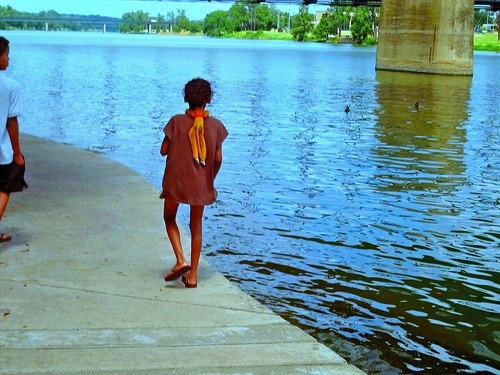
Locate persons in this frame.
[159,78,229,287]
[0,36,28,244]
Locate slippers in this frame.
[181,271,198,288]
[164,264,192,282]
[1,233,12,243]
